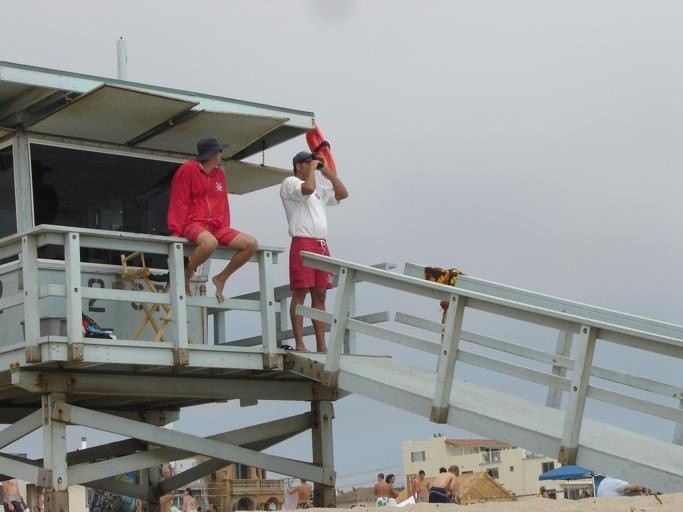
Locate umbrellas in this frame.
[537,464,595,498]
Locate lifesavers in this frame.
[306,127,336,179]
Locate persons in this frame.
[279,151,350,352]
[134,496,142,511]
[538,485,549,499]
[427,464,461,504]
[1,478,26,512]
[372,473,389,507]
[167,136,258,303]
[159,491,177,511]
[438,466,447,473]
[411,469,432,502]
[181,486,198,512]
[285,478,314,508]
[384,472,400,507]
[595,476,643,496]
[161,462,171,480]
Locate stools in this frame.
[119,251,197,343]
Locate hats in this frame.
[291,151,318,166]
[192,137,229,162]
